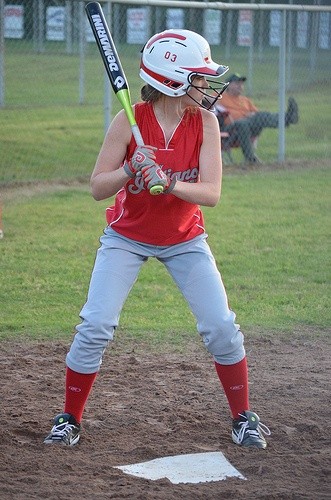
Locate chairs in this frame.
[221,131,240,164]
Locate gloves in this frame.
[123,144,158,177]
[136,163,176,194]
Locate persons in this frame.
[211,73,298,167]
[42,29,271,450]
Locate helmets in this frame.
[139,28,229,97]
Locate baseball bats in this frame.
[84,1,164,196]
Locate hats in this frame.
[226,74,247,83]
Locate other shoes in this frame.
[286,98,298,126]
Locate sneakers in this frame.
[42,413,80,447]
[230,411,271,448]
